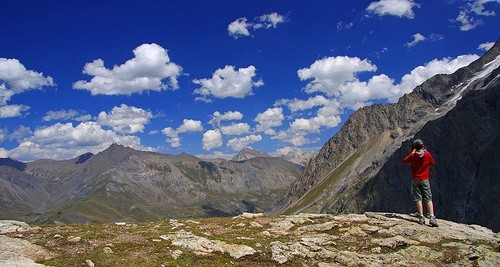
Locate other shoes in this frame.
[419,215,425,225]
[410,212,418,217]
[429,215,439,227]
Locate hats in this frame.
[413,139,423,147]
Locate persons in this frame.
[402,139,439,226]
